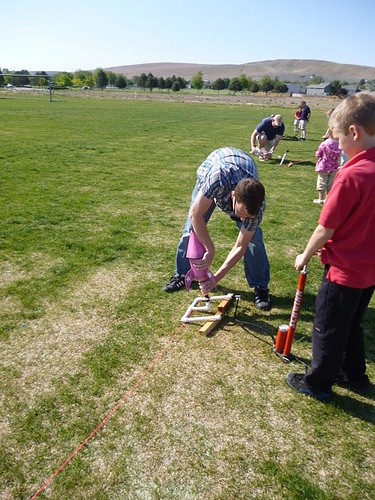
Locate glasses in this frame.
[234,198,258,220]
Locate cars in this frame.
[82,86,93,89]
[5,83,14,89]
[25,85,32,88]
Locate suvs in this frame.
[48,82,70,90]
[105,85,119,89]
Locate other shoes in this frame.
[313,199,326,204]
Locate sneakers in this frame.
[164,276,191,293]
[335,374,370,391]
[255,290,271,310]
[288,373,332,400]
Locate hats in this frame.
[274,115,283,126]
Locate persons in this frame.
[251,114,285,158]
[313,128,341,204]
[327,108,346,169]
[294,100,311,140]
[163,148,272,311]
[285,90,375,400]
